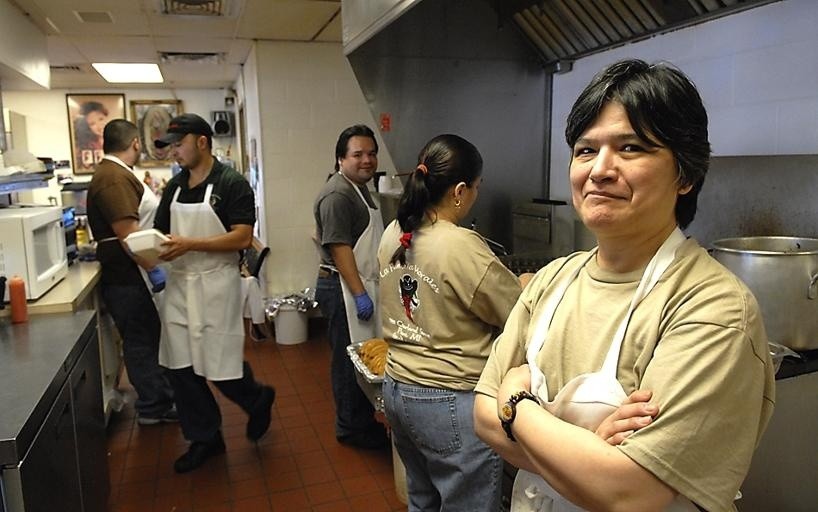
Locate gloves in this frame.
[146,265,166,293]
[353,290,374,322]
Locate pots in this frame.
[707,236,818,350]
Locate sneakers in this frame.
[245,386,275,442]
[135,403,184,426]
[338,422,390,448]
[173,430,227,474]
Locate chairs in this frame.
[237,235,269,343]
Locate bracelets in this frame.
[496,389,541,442]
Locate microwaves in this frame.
[0,208,68,303]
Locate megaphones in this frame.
[222,88,236,107]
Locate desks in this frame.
[0,259,125,430]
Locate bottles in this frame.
[144,171,152,189]
[0,274,28,324]
[74,217,89,251]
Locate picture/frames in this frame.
[129,100,185,169]
[66,93,126,175]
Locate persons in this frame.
[376,131,538,511]
[72,101,115,171]
[468,57,777,511]
[310,123,391,451]
[149,111,276,474]
[83,117,179,427]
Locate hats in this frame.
[154,114,214,149]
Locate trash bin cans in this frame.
[275,304,308,345]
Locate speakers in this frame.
[211,111,234,137]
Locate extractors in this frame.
[497,0,818,73]
